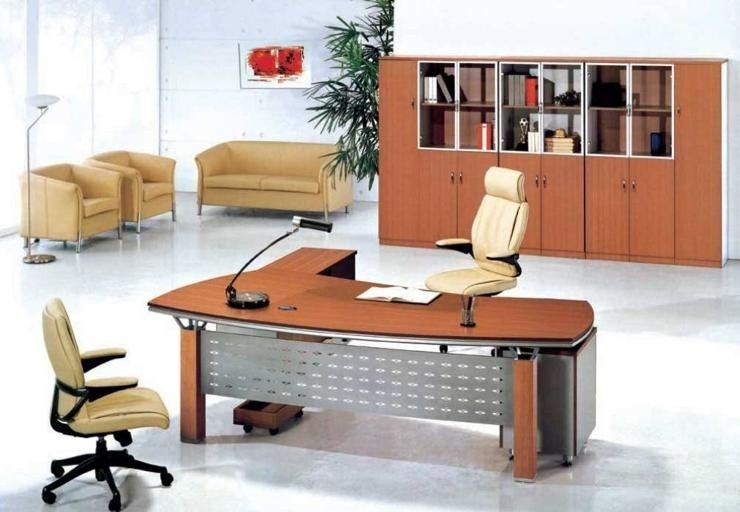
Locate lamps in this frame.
[224,213,333,309]
[22,93,60,265]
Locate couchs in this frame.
[193,139,354,221]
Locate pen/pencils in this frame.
[278,306,298,310]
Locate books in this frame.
[423,73,581,153]
[354,285,442,305]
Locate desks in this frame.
[148,246,594,486]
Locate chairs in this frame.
[424,166,531,298]
[41,297,173,512]
[18,149,177,254]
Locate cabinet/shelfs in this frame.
[418,56,673,159]
[495,326,598,469]
[673,56,730,267]
[417,147,675,264]
[377,55,417,246]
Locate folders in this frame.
[423,74,454,105]
